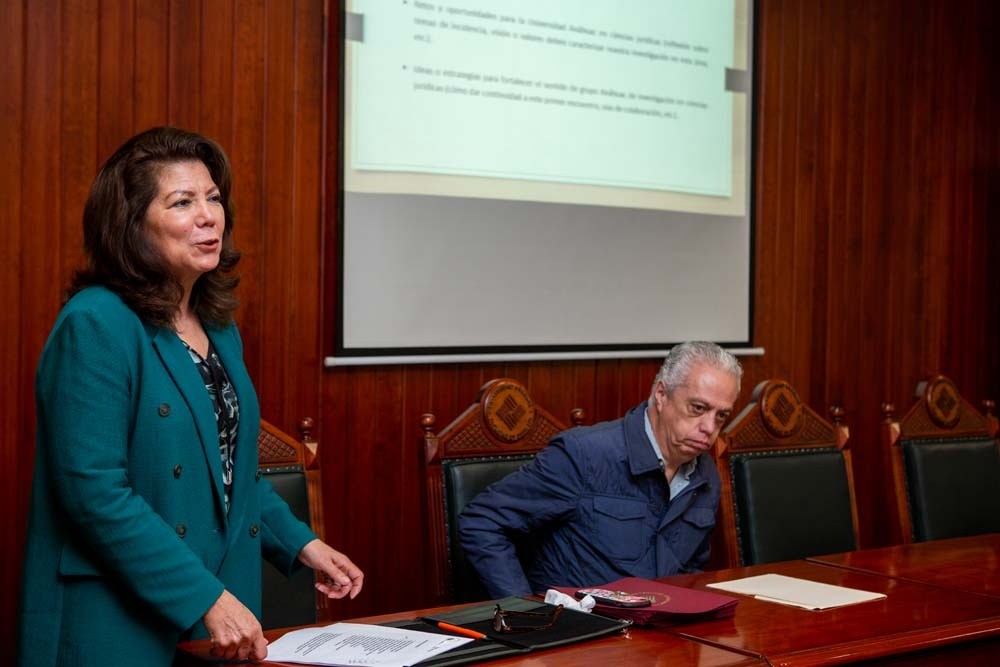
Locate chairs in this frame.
[420,378,586,607]
[259,414,332,630]
[715,379,860,567]
[881,374,1000,545]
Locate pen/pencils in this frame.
[437,622,488,639]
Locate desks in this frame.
[179,534,1000,667]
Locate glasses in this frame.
[493,603,564,632]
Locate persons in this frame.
[455,340,742,600]
[10,127,364,667]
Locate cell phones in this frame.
[574,588,651,608]
